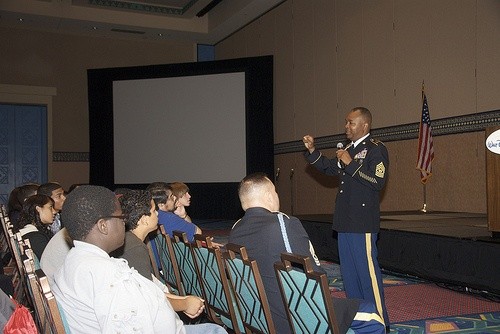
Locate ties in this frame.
[348,145,354,152]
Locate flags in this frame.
[415,93,437,185]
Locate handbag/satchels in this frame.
[4,306,36,333]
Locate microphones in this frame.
[275,167,280,180]
[337,143,343,168]
[290,169,294,179]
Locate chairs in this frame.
[0,205,344,334]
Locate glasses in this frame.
[95,214,129,224]
[157,193,175,204]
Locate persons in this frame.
[50,186,228,334]
[228,172,360,334]
[109,190,205,319]
[17,184,38,206]
[303,107,390,334]
[7,187,21,231]
[40,227,75,292]
[147,182,202,274]
[169,182,224,249]
[18,195,56,262]
[114,188,130,199]
[37,183,66,235]
